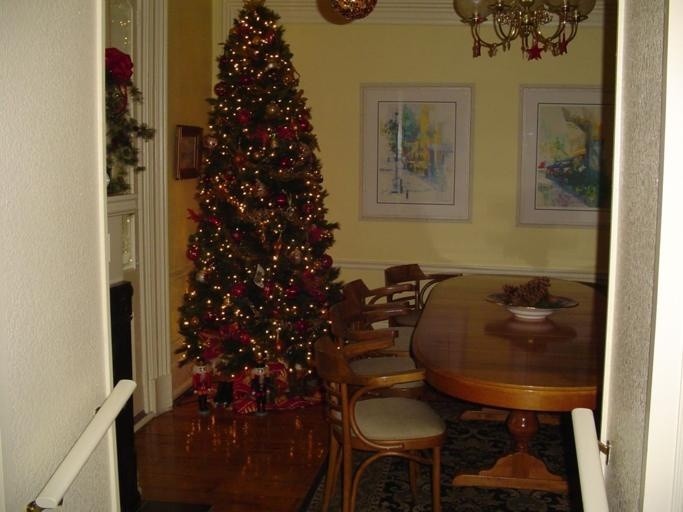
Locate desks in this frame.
[410,273,605,497]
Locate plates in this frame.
[484,294,579,318]
[483,316,577,343]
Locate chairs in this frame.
[311,260,461,511]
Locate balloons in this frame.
[328,0,377,20]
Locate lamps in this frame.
[450,0,595,63]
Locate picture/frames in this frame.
[172,124,204,181]
[514,81,612,230]
[356,82,474,224]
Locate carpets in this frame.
[295,398,584,511]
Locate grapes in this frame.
[497,275,554,312]
